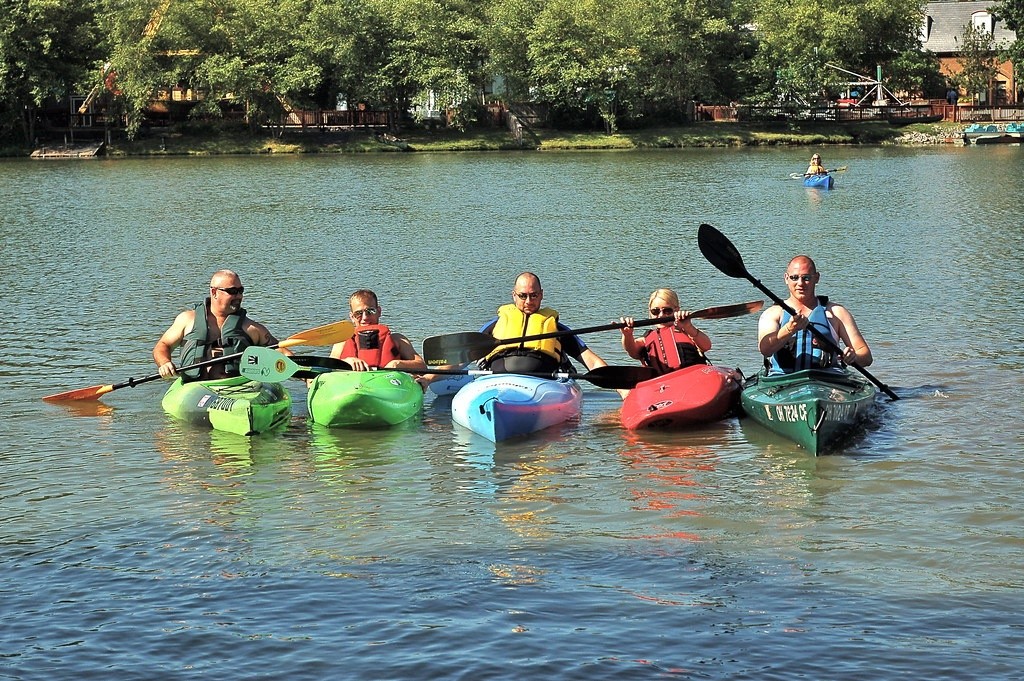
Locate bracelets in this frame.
[784,324,795,336]
[689,328,699,337]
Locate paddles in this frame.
[41,319,355,401]
[286,356,659,389]
[697,224,900,400]
[423,301,766,366]
[239,346,475,396]
[789,166,847,180]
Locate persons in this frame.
[152,268,317,388]
[757,254,873,375]
[609,289,712,369]
[327,289,429,375]
[414,272,631,401]
[804,153,829,178]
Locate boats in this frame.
[620,364,742,432]
[451,372,584,445]
[958,122,1024,144]
[306,370,423,430]
[429,376,475,396]
[162,376,293,437]
[804,174,834,189]
[741,363,879,459]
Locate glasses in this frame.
[786,275,816,281]
[514,292,541,300]
[649,307,677,315]
[352,307,378,319]
[813,158,820,160]
[212,286,244,296]
